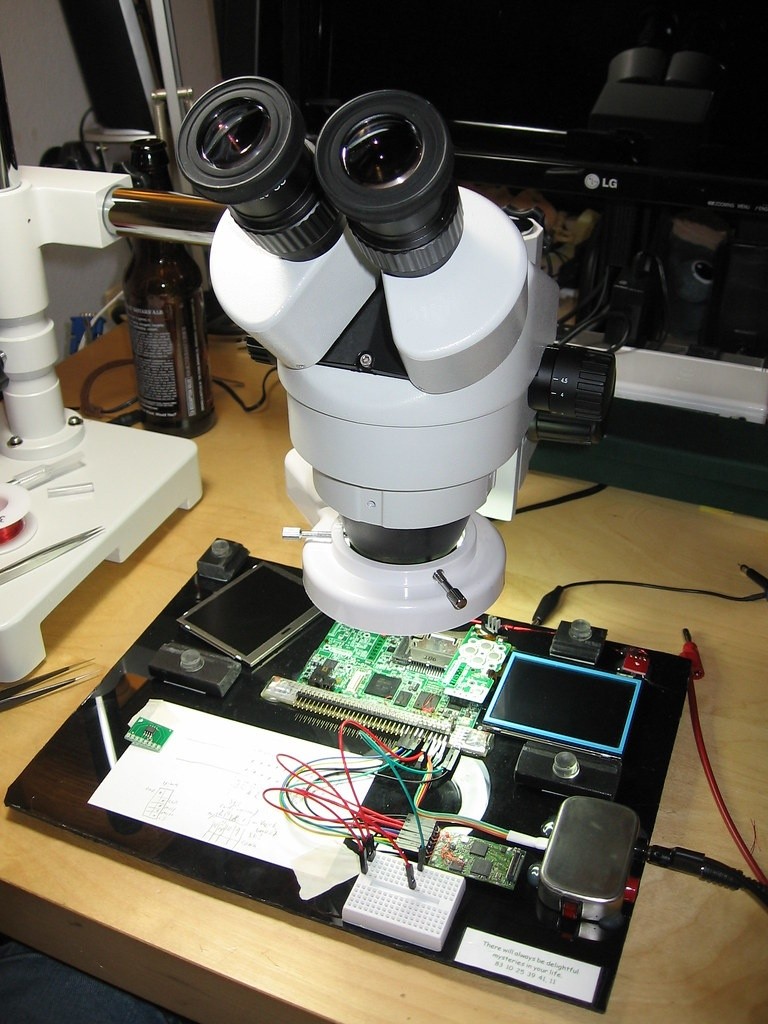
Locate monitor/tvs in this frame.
[210,0,768,222]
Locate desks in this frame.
[0,318,768,1023]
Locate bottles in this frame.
[123,140,217,441]
